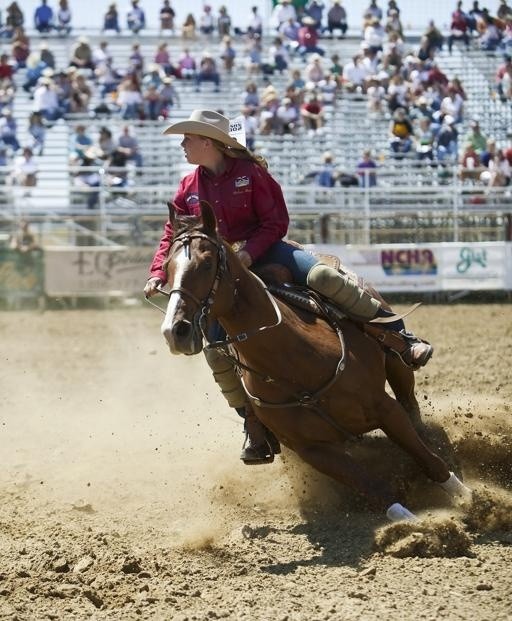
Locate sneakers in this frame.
[400,327,435,368]
[239,415,280,463]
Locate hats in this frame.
[162,106,250,153]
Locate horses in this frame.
[160,200,474,526]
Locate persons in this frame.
[0,0,511,245]
[8,218,44,279]
[141,105,434,465]
